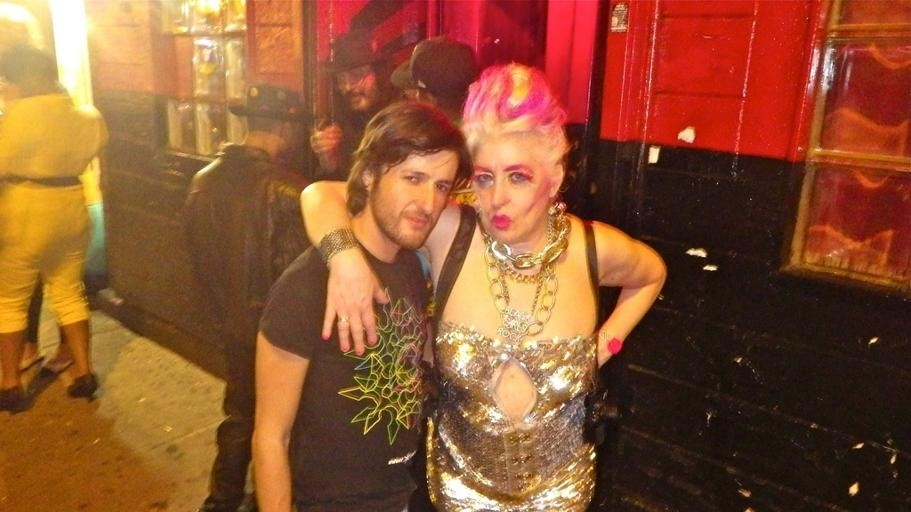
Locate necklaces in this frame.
[474,202,574,343]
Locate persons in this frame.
[242,100,474,511]
[385,36,482,129]
[297,59,668,512]
[0,47,113,420]
[307,26,414,183]
[175,83,324,512]
[0,16,79,378]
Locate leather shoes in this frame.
[40,352,74,374]
[19,347,48,372]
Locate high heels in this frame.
[65,378,99,400]
[0,385,22,411]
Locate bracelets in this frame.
[596,325,625,356]
[315,223,362,273]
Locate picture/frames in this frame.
[780,3,911,288]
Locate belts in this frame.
[8,175,86,189]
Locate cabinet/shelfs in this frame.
[85,2,306,373]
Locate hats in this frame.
[228,82,314,121]
[318,35,386,74]
[389,36,480,98]
